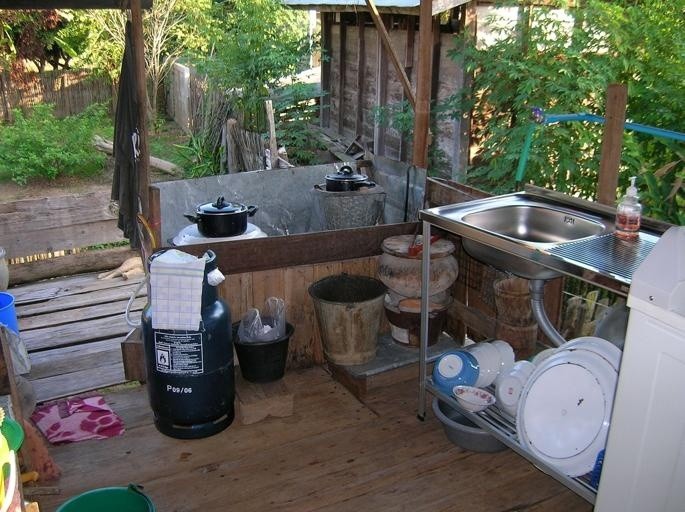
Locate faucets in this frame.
[513,108,546,182]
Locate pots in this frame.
[183,196,258,237]
[314,165,376,192]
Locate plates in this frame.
[495,361,538,417]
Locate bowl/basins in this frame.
[454,385,496,413]
[432,339,516,391]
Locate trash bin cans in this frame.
[232,315,295,383]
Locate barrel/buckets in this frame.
[309,273,387,366]
[53,481,156,512]
[0,430,17,512]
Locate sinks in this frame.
[460,202,606,246]
